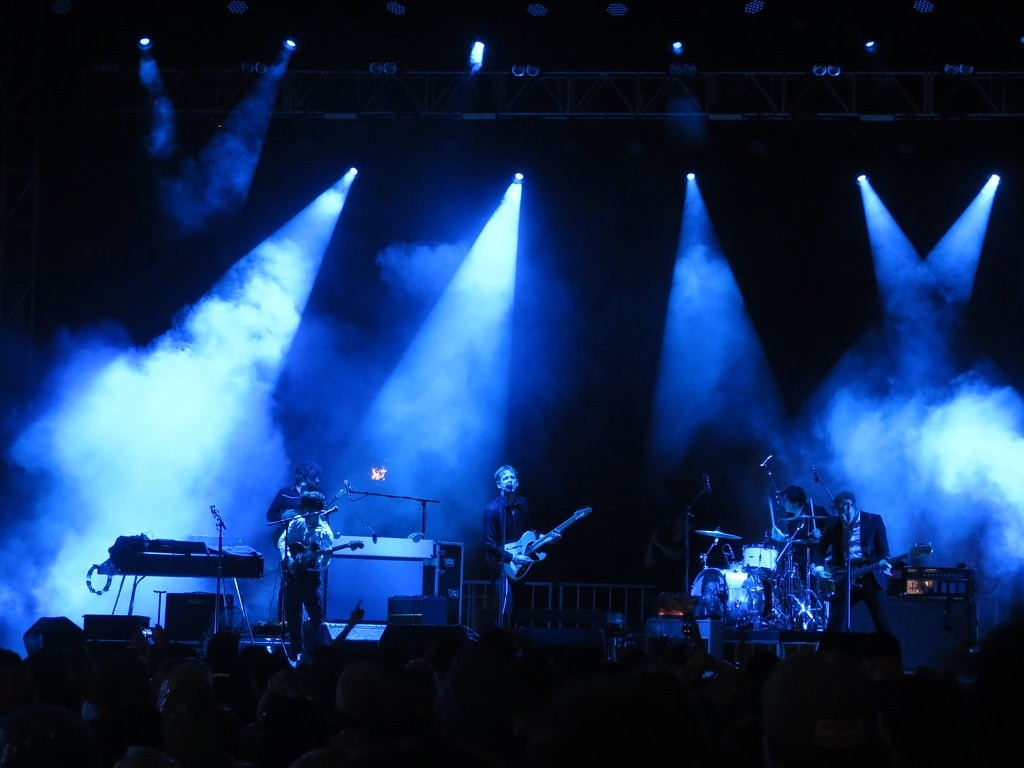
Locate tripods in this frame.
[758,519,826,631]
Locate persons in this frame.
[266,462,329,625]
[278,492,334,661]
[483,465,562,631]
[0,577,1024,768]
[817,491,890,631]
[781,485,832,580]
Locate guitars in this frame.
[282,540,365,575]
[273,485,353,548]
[819,541,934,600]
[502,505,593,582]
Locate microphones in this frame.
[209,504,217,518]
[760,455,772,467]
[846,500,853,505]
[321,506,338,516]
[812,465,819,483]
[505,483,512,489]
[344,479,351,498]
[727,544,735,558]
[706,476,712,494]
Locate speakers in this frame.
[517,626,607,659]
[165,594,234,641]
[814,633,903,678]
[307,622,387,646]
[24,616,90,657]
[376,598,469,656]
[646,618,724,661]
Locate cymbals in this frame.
[791,537,819,545]
[693,529,742,540]
[778,514,830,521]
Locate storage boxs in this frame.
[83,615,149,640]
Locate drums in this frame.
[690,567,764,626]
[771,562,799,579]
[742,544,780,575]
[728,561,743,570]
